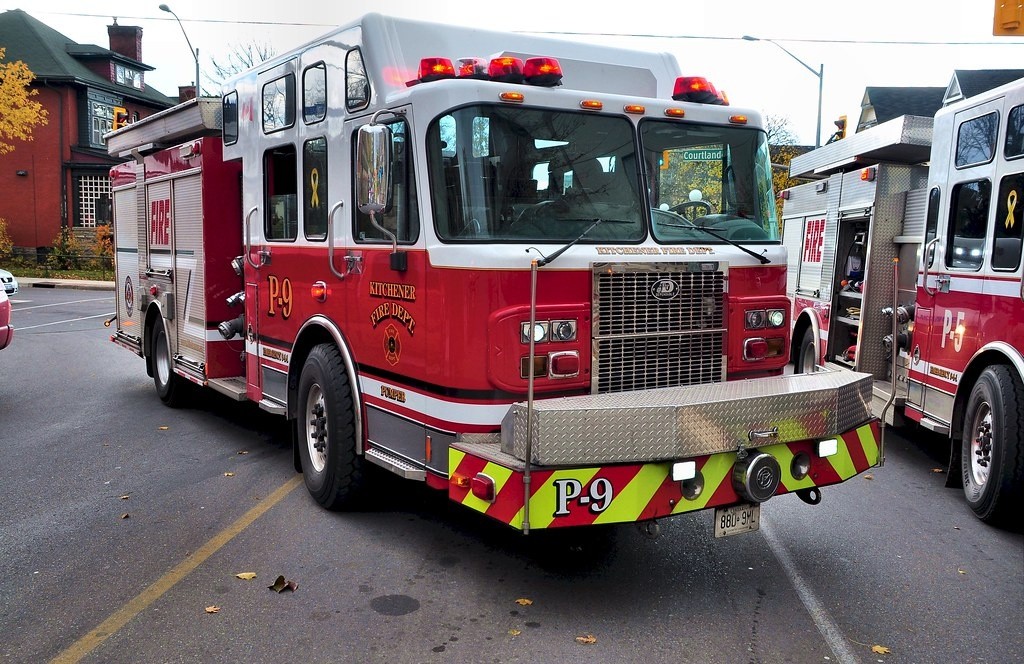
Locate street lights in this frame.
[741,36,824,150]
[159,4,200,98]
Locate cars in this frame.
[0,269,18,297]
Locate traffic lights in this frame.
[833,116,846,141]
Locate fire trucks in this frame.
[101,13,888,535]
[779,74,1024,530]
